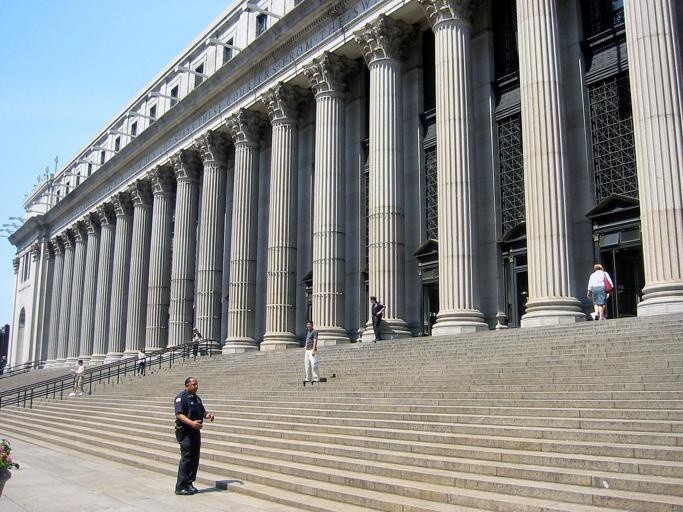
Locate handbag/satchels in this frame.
[605,277,612,293]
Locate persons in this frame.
[135,348,146,377]
[370,295,385,342]
[586,264,614,320]
[190,327,202,359]
[302,321,320,382]
[173,377,214,495]
[68,360,85,397]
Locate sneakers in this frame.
[69,392,85,397]
[591,312,606,320]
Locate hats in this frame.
[594,263,602,268]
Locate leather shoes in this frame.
[175,484,197,495]
